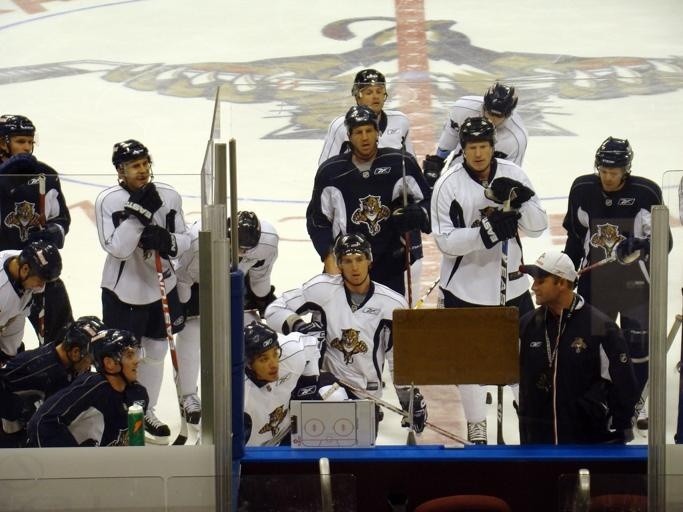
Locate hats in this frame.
[519,251,577,283]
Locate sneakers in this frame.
[637,407,648,431]
[180,395,201,424]
[142,413,171,437]
[467,420,487,442]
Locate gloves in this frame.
[490,177,536,210]
[479,208,522,250]
[0,152,36,173]
[291,375,318,399]
[616,237,650,266]
[393,205,430,233]
[138,225,177,259]
[294,320,324,334]
[124,182,163,227]
[422,154,446,188]
[29,223,64,249]
[401,388,429,434]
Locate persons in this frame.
[0,115,280,446]
[239,70,673,448]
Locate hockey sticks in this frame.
[153,253,188,446]
[401,129,412,312]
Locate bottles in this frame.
[127,404,144,445]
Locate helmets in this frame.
[351,69,386,97]
[227,211,261,251]
[345,105,378,133]
[459,116,496,148]
[62,315,144,369]
[334,233,372,260]
[0,114,35,137]
[484,83,518,118]
[112,140,148,163]
[245,321,278,357]
[595,136,634,169]
[18,240,62,282]
